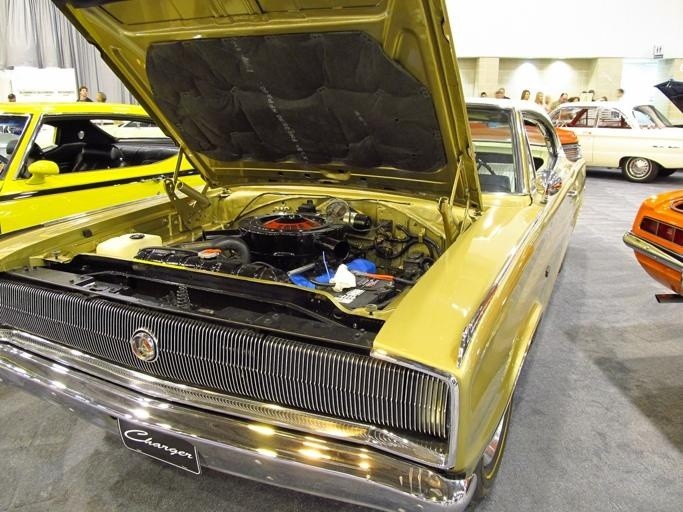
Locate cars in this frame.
[0,122,24,165]
[651,78,683,128]
[467,118,580,162]
[0,100,208,254]
[547,102,682,183]
[99,119,177,147]
[0,3,584,512]
[620,190,682,304]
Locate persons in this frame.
[76,86,92,101]
[96,91,106,102]
[480,88,624,113]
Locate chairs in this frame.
[4,138,45,179]
[71,143,127,172]
